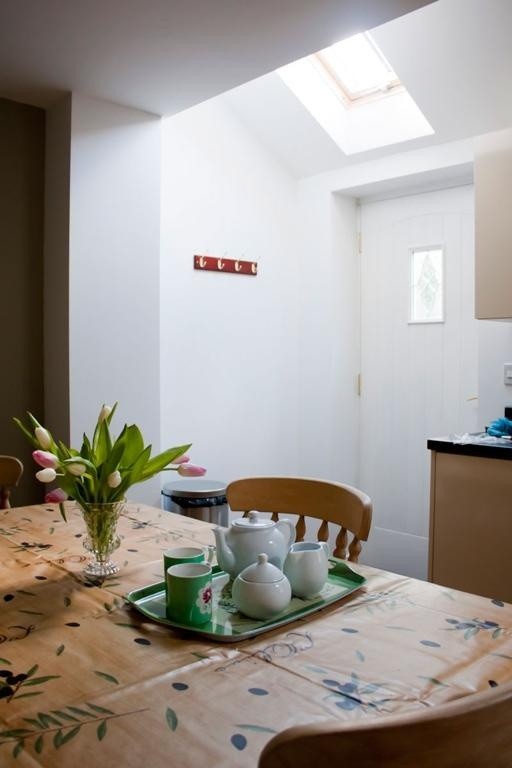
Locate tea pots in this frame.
[212,510,295,576]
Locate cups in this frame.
[162,544,216,628]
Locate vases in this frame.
[75,497,127,576]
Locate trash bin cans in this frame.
[160,479,229,528]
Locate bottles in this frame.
[284,540,331,597]
[230,554,291,620]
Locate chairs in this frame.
[255,685,509,768]
[227,473,370,570]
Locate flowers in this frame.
[13,403,209,554]
